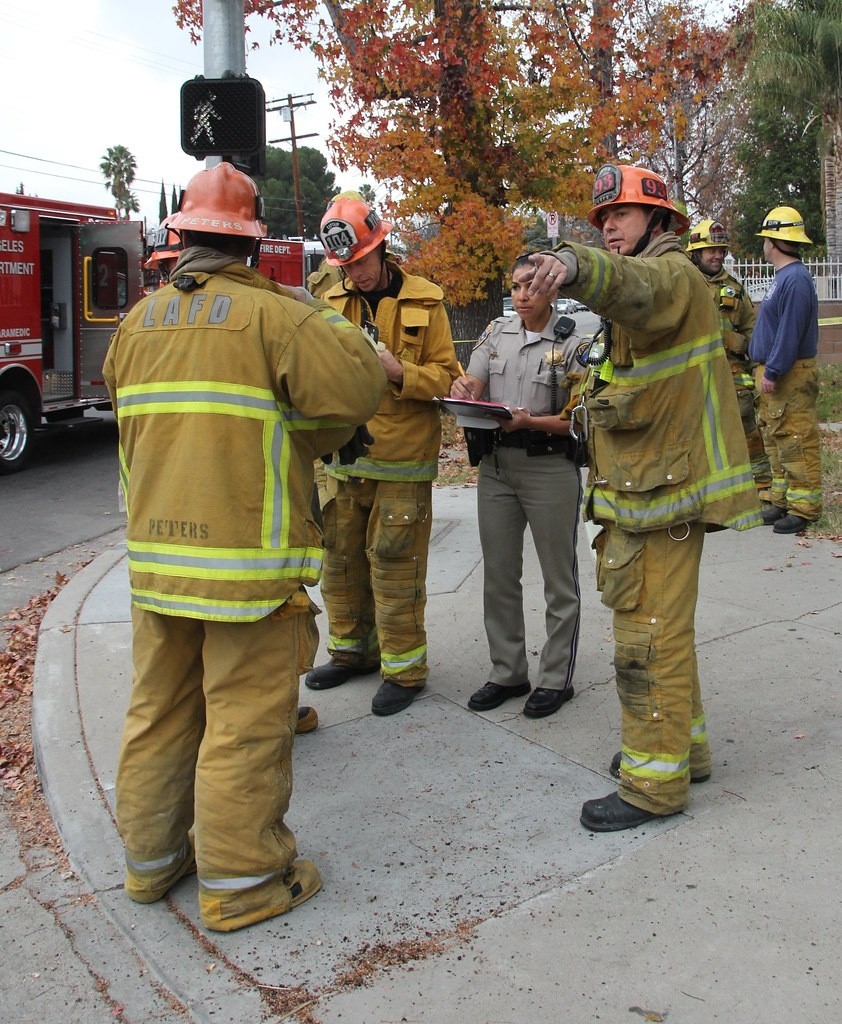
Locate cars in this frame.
[504,297,517,317]
[551,298,590,314]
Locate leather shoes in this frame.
[760,506,788,525]
[371,679,425,716]
[580,791,684,831]
[305,655,381,690]
[609,751,711,784]
[523,685,574,718]
[773,514,822,534]
[468,680,531,711]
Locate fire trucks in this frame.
[0,192,156,476]
[257,238,325,288]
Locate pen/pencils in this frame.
[457,361,475,402]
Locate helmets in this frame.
[686,220,732,252]
[755,206,813,245]
[143,212,183,269]
[325,191,366,210]
[587,163,690,236]
[166,162,268,238]
[319,197,394,267]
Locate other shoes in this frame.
[200,859,323,932]
[294,706,318,733]
[125,828,197,904]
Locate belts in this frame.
[495,427,530,450]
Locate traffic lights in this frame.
[180,72,266,158]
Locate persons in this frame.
[450,250,595,719]
[306,190,402,299]
[517,164,765,834]
[685,219,774,511]
[747,206,824,534]
[101,162,390,933]
[304,198,461,717]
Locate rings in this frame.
[547,272,556,280]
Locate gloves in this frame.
[320,425,376,466]
